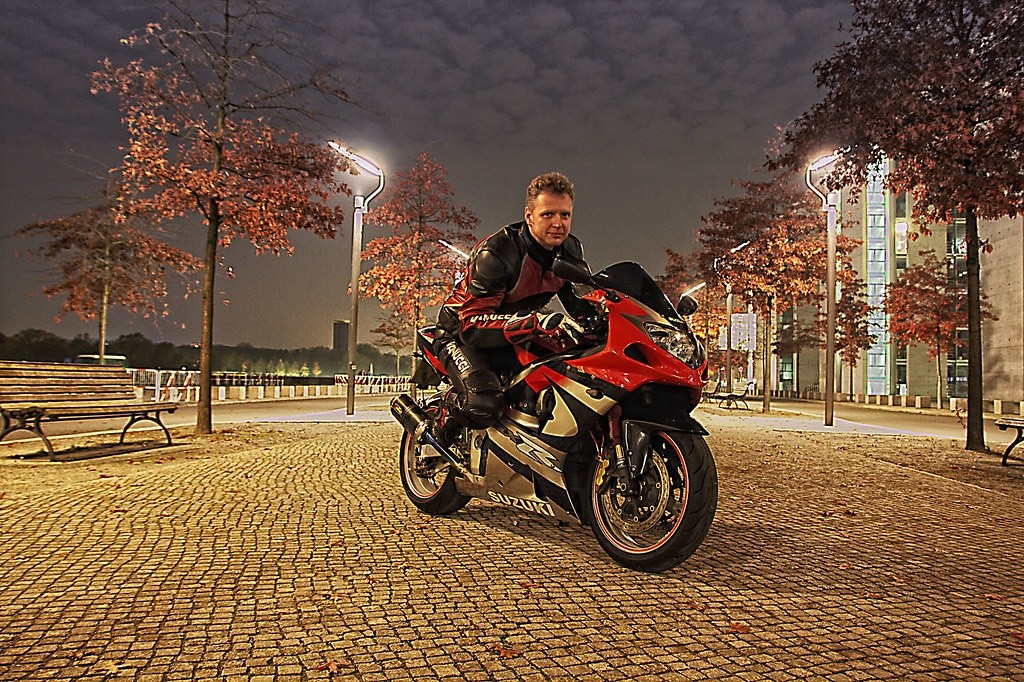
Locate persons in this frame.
[433,172,592,446]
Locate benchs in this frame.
[993,417,1024,466]
[0,359,179,462]
[717,381,754,410]
[700,381,722,404]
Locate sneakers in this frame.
[430,384,457,434]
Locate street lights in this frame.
[327,140,385,416]
[712,240,750,408]
[805,146,855,428]
[679,282,709,333]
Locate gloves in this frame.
[505,308,583,350]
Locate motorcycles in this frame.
[388,261,718,575]
